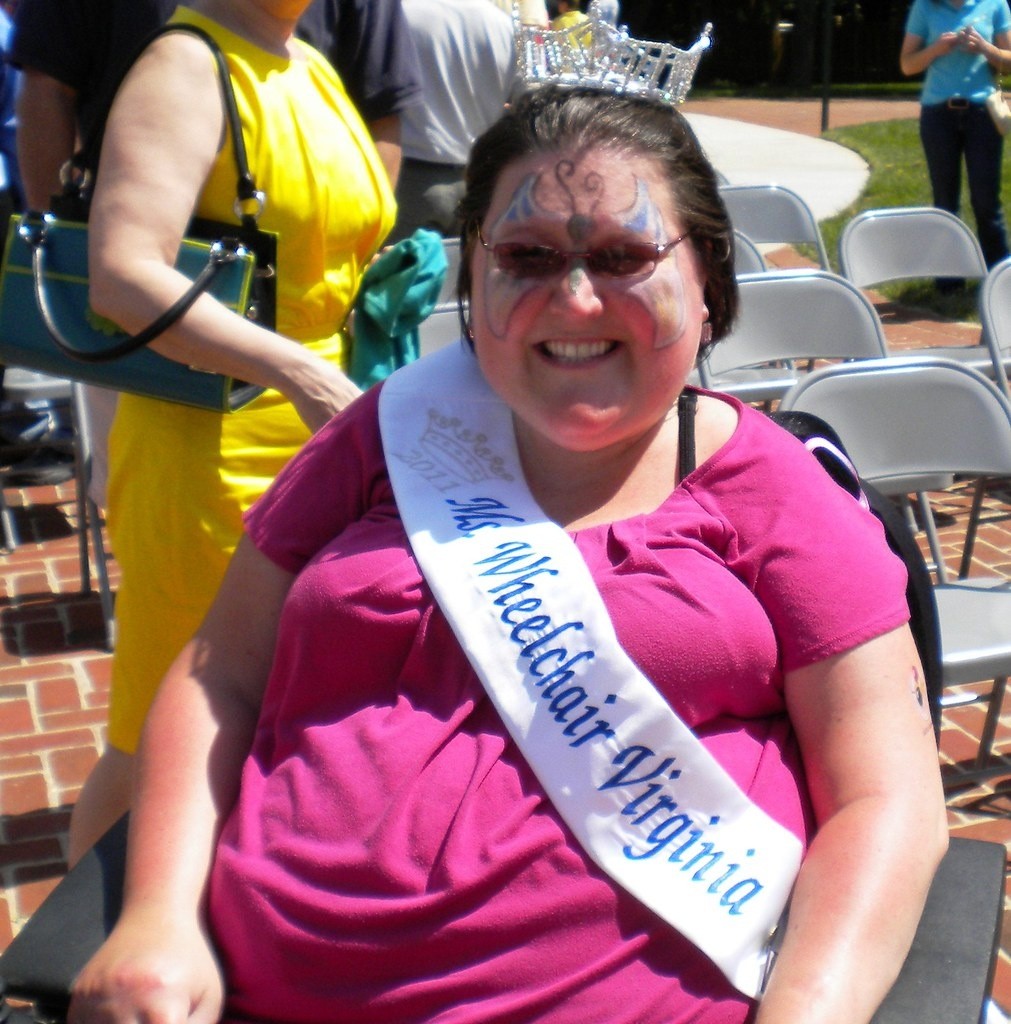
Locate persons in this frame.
[901,0,1011,290]
[5,1,184,225]
[67,0,400,871]
[292,1,418,191]
[380,0,549,251]
[66,83,952,1024]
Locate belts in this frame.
[944,99,977,109]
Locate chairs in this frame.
[686,230,811,416]
[960,256,1011,584]
[419,300,470,359]
[713,185,854,420]
[777,356,1011,789]
[692,265,949,584]
[0,477,1006,1023]
[73,384,119,655]
[838,206,1011,537]
[433,239,470,305]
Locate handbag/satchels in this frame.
[985,49,1011,136]
[0,23,277,414]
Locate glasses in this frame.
[474,218,701,279]
